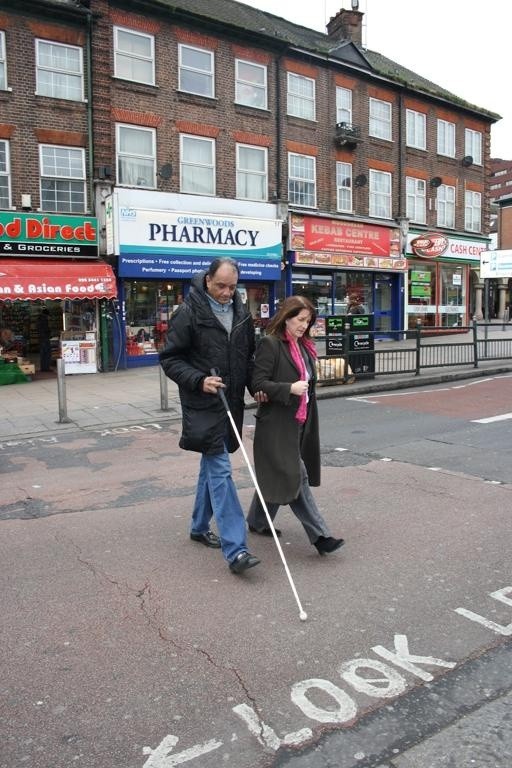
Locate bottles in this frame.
[76,304,80,316]
[89,320,92,331]
[69,327,81,340]
[0,302,24,335]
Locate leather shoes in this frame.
[247,522,281,537]
[312,534,345,556]
[190,529,222,548]
[229,550,261,574]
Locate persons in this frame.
[36,308,51,372]
[247,296,345,556]
[158,258,267,575]
